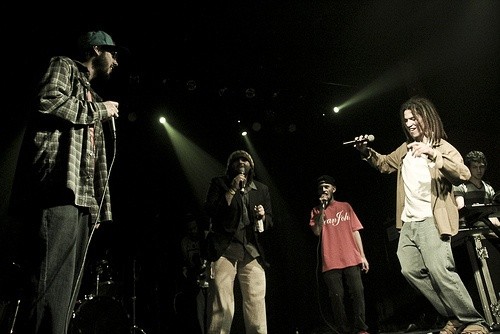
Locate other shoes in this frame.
[460,324,489,334]
[440,319,465,334]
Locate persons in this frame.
[354,97,489,334]
[309,175,370,334]
[453,150,500,227]
[179,211,205,334]
[6,30,120,334]
[200,149,273,334]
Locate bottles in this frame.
[253,203,264,233]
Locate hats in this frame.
[225,150,255,176]
[85,30,116,47]
[316,174,338,186]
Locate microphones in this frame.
[343,134,375,145]
[238,168,246,193]
[322,191,328,209]
[109,116,116,139]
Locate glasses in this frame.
[233,157,249,161]
[103,48,118,59]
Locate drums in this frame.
[74,295,131,334]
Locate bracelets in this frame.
[228,187,236,195]
[429,148,437,162]
